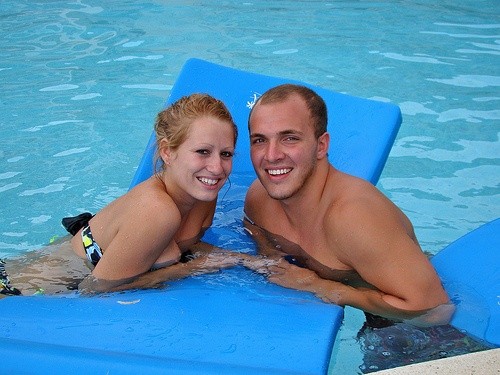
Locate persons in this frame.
[243,83,456,326]
[0,93,288,298]
